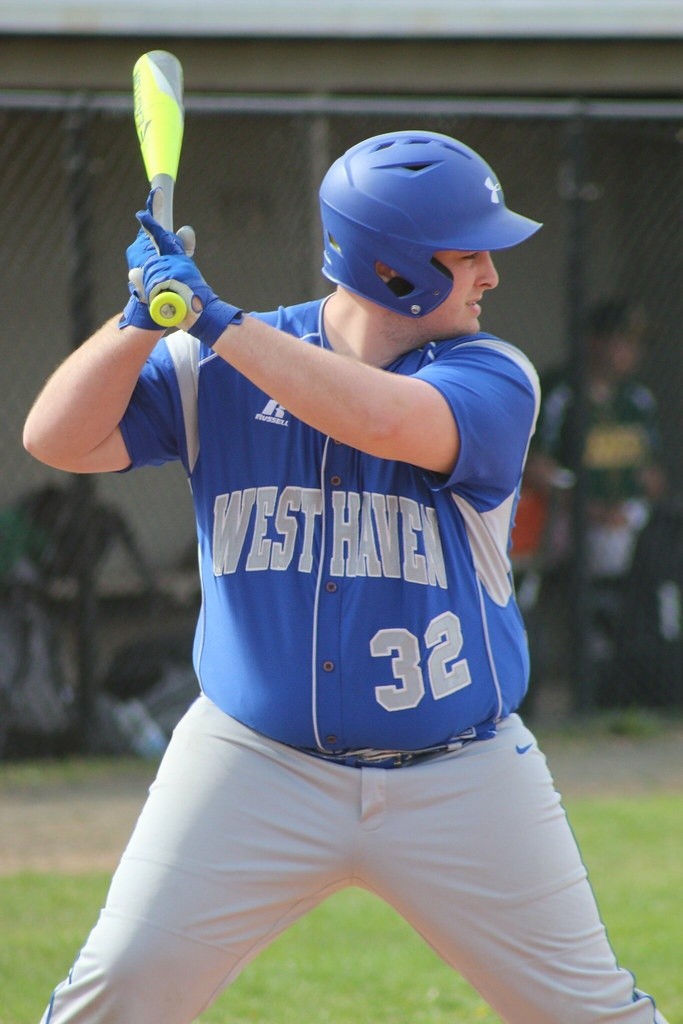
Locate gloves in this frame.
[118,186,245,348]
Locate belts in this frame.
[296,716,498,769]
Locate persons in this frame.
[21,129,669,1023]
[519,296,661,719]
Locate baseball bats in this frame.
[132,49,186,329]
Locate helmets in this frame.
[318,130,543,318]
